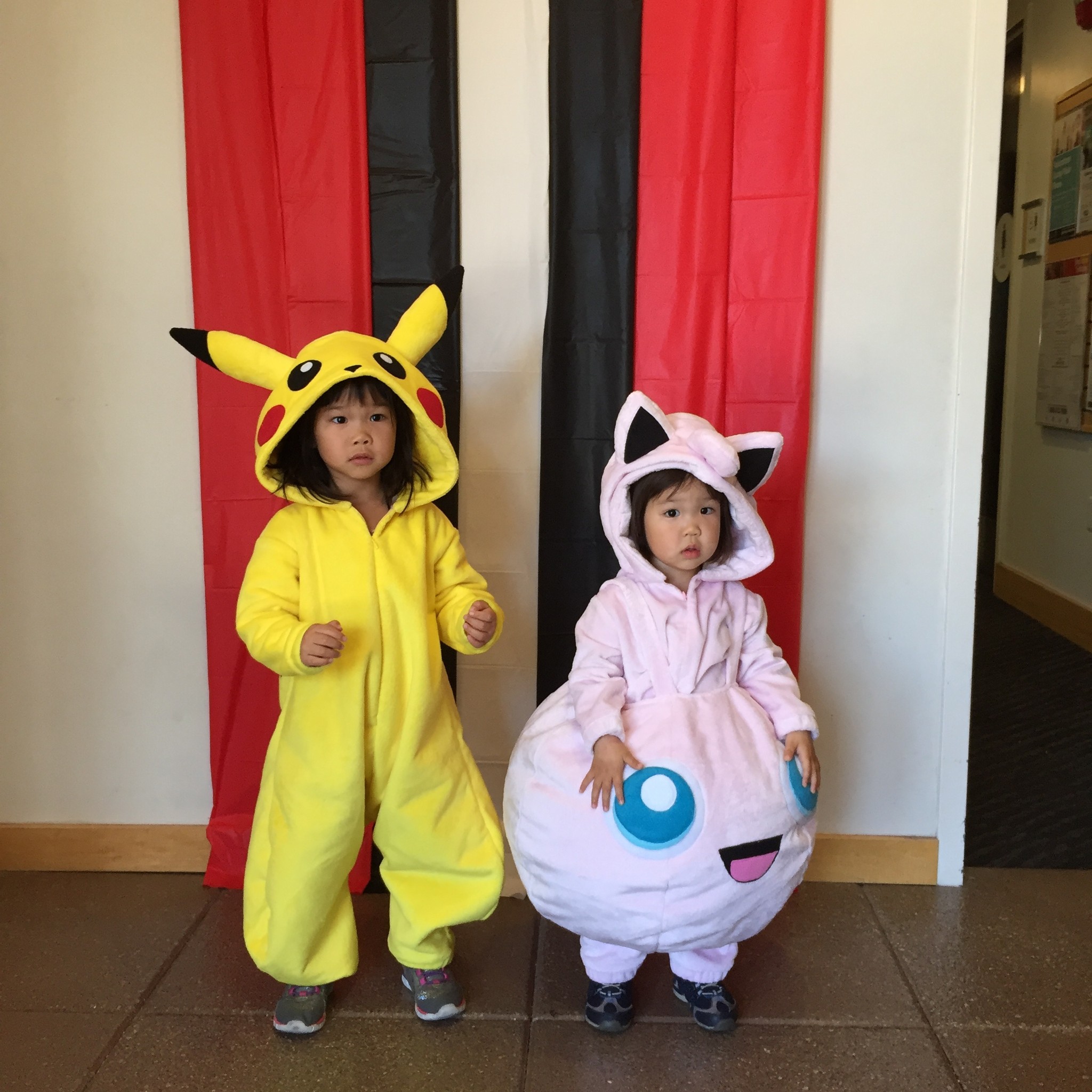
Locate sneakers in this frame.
[271,982,333,1036]
[673,974,741,1032]
[585,979,636,1036]
[398,965,467,1021]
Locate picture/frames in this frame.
[1044,78,1092,264]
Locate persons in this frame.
[166,265,504,1037]
[502,388,822,1034]
